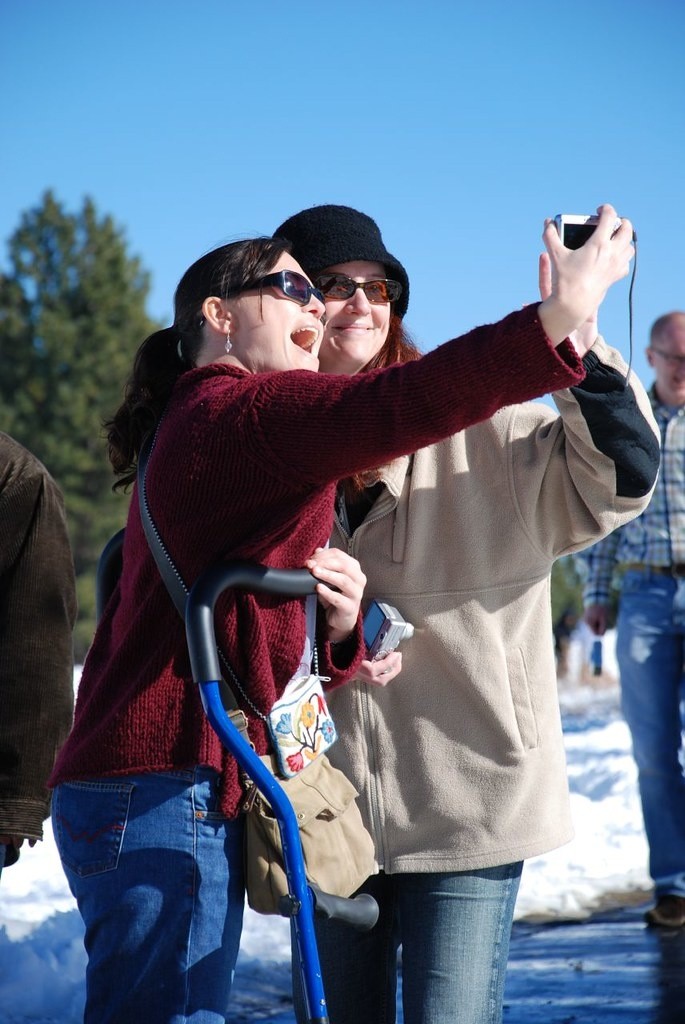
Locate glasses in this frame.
[197,270,326,331]
[651,347,685,366]
[312,272,403,303]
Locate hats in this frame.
[273,205,409,321]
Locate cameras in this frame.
[361,599,415,674]
[553,214,621,249]
[590,642,602,676]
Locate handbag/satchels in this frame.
[241,753,378,918]
[266,676,340,779]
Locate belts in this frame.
[629,563,685,582]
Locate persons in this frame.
[274,205,685,1024]
[0,427,75,877]
[46,204,636,1024]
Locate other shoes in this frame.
[645,895,685,927]
